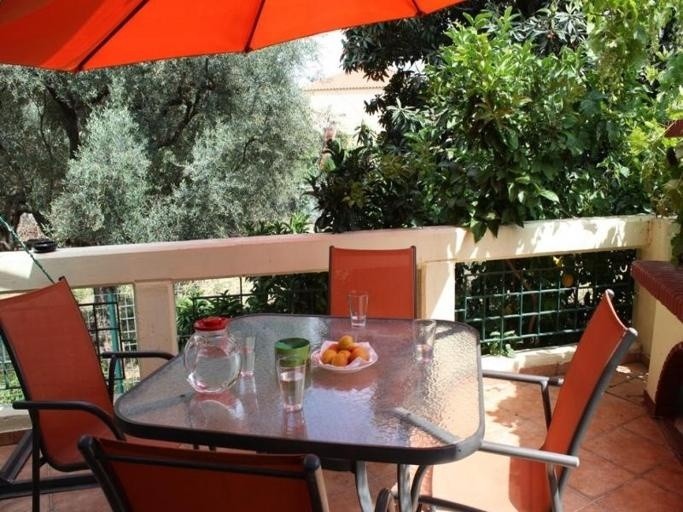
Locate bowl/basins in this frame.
[274,337,311,365]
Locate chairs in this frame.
[78,433,330,512]
[376,289,638,512]
[1,276,174,511]
[328,245,417,321]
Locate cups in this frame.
[347,290,367,328]
[277,357,306,414]
[411,319,436,362]
[236,330,256,377]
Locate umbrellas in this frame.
[0,1,464,72]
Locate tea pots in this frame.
[182,318,242,396]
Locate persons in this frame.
[320,128,340,167]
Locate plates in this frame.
[32,239,59,252]
[314,340,378,375]
